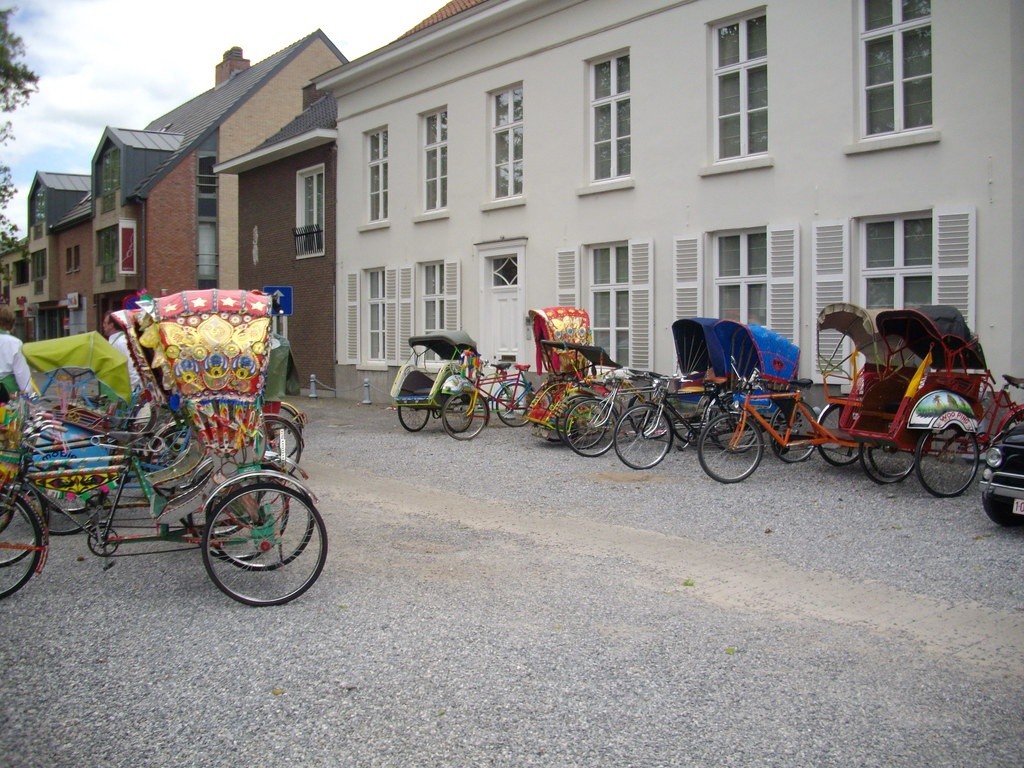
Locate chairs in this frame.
[413,387,432,394]
[680,387,707,397]
[828,395,863,408]
[863,399,898,420]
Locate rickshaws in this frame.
[389,303,1024,499]
[0,287,329,609]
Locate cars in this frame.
[977,421,1024,529]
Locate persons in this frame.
[0,309,36,398]
[102,311,140,392]
